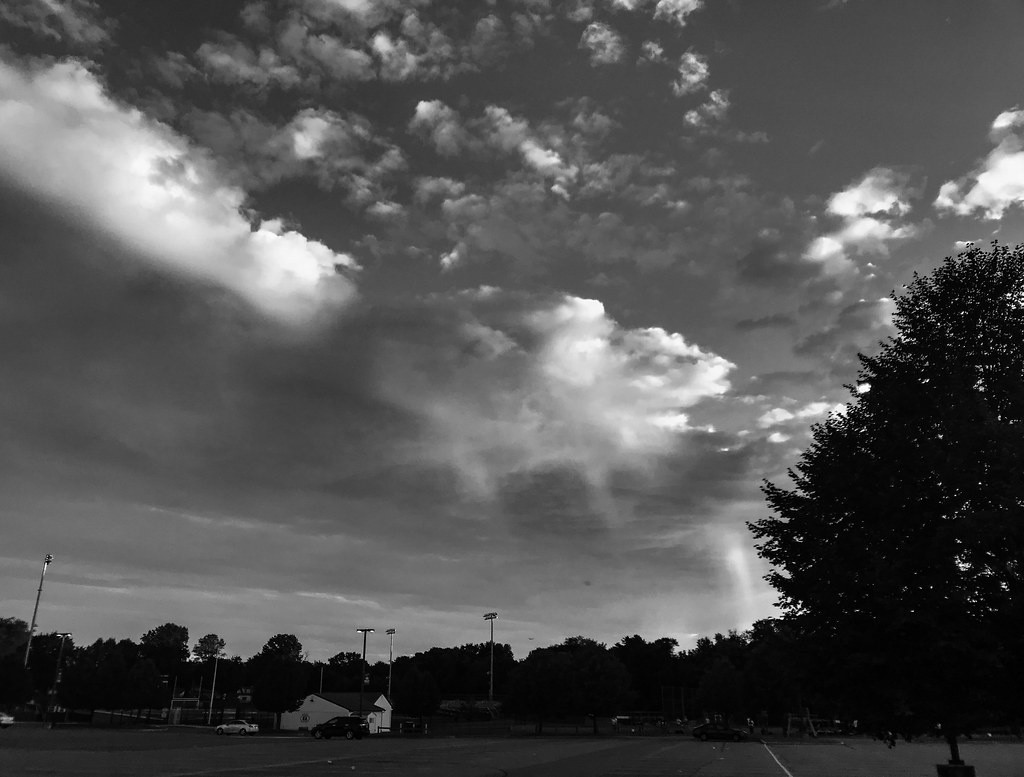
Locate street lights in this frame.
[207,641,226,723]
[23,554,54,667]
[356,627,376,718]
[483,612,500,702]
[384,627,397,703]
[44,632,73,723]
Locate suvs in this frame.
[310,715,370,740]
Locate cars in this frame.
[215,719,259,737]
[0,712,14,728]
[692,722,749,742]
